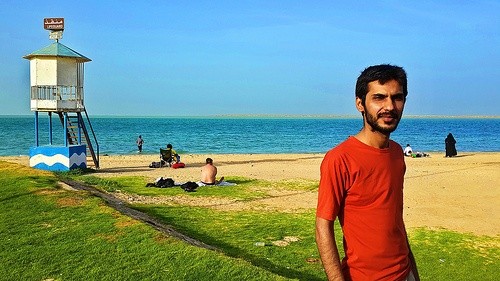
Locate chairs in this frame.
[159,148,180,166]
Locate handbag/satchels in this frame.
[148,161,160,168]
[156,177,174,187]
[181,181,199,191]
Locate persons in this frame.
[136,135,144,153]
[408,150,429,158]
[403,143,413,156]
[200,158,224,185]
[161,144,178,166]
[313,65,419,281]
[445,134,456,157]
